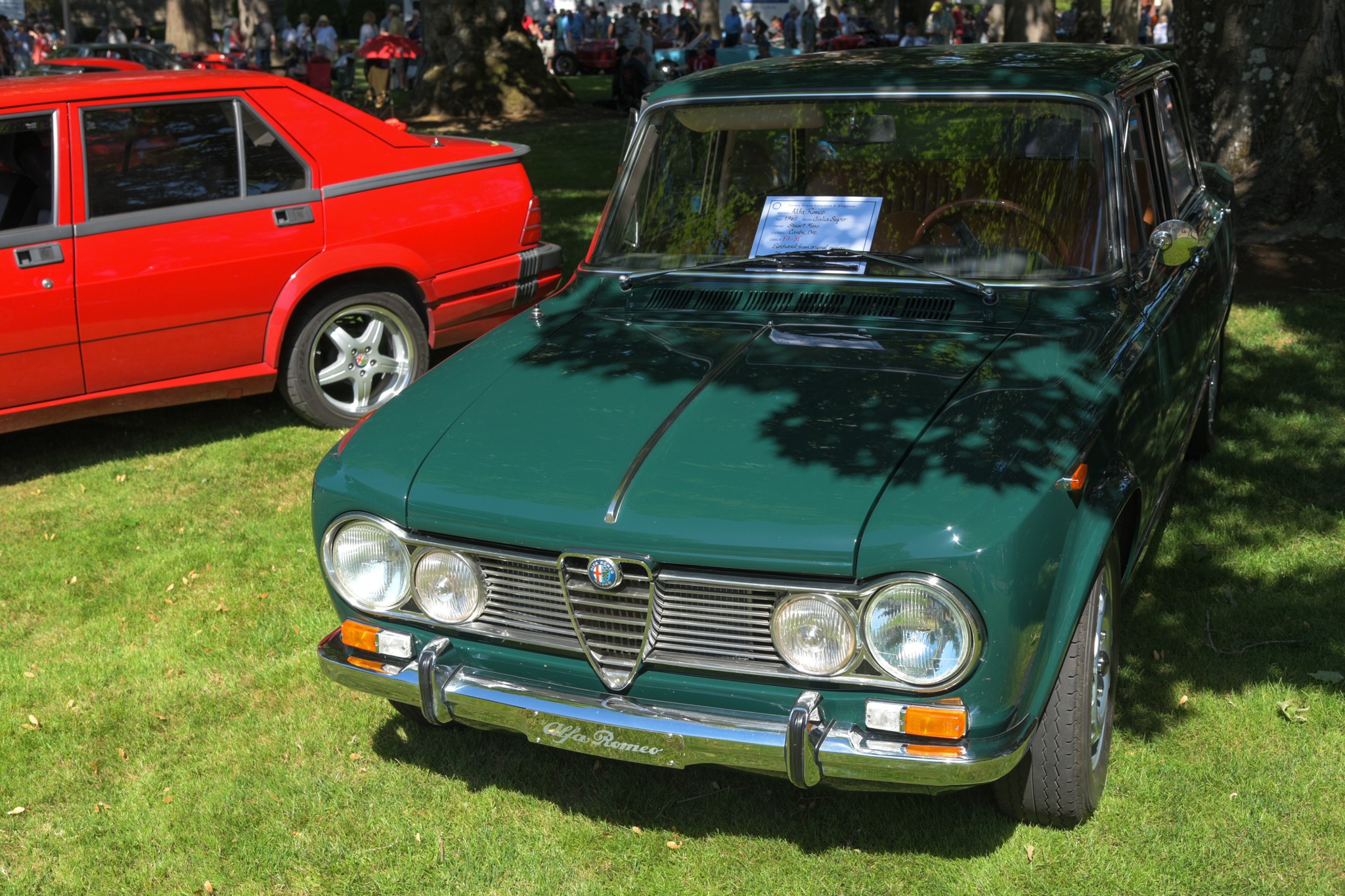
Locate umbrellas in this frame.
[357,35,426,119]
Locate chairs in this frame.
[724,158,1154,275]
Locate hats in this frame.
[695,40,707,47]
[930,1,943,13]
[615,45,631,57]
[628,3,645,11]
[905,22,917,27]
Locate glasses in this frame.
[697,47,704,49]
[908,27,917,29]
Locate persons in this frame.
[854,1,1170,48]
[521,1,852,121]
[0,9,153,79]
[213,11,355,102]
[378,4,424,89]
[360,10,379,84]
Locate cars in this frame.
[653,30,804,77]
[0,63,567,439]
[305,40,1240,831]
[550,27,682,77]
[16,38,245,81]
